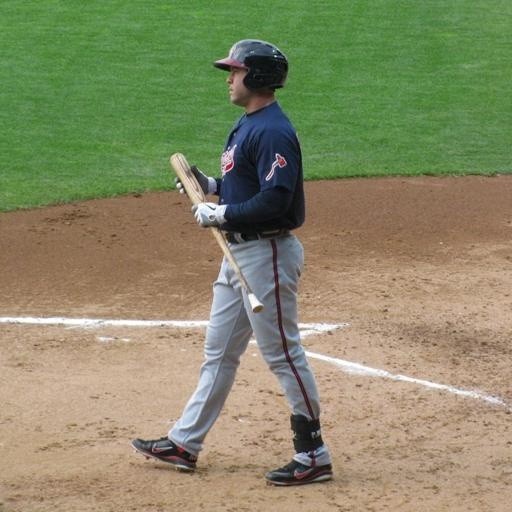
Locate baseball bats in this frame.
[170,153,264,313]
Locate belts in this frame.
[225,227,289,243]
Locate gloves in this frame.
[191,202,229,228]
[174,164,217,199]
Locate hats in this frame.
[213,39,288,91]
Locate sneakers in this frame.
[264,460,332,486]
[131,436,198,472]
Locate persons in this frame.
[127,38,337,489]
